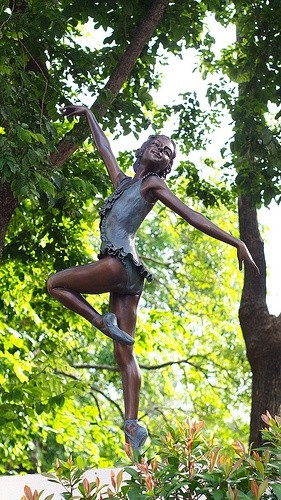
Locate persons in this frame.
[44,105,264,467]
